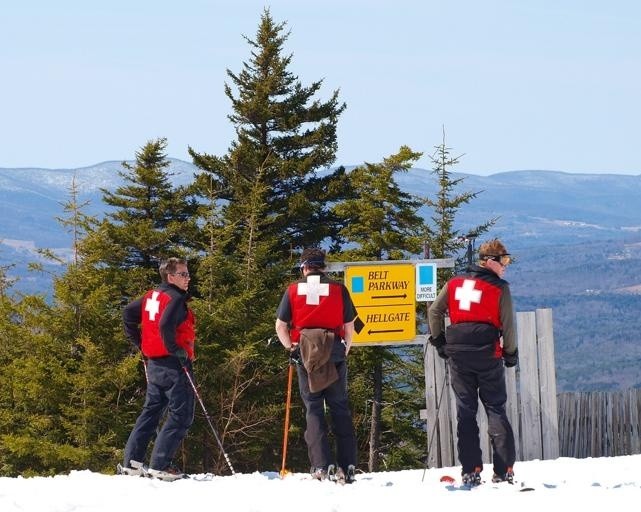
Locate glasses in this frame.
[172,271,191,278]
[497,253,510,266]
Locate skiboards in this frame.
[440,476,557,491]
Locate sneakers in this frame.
[149,464,184,479]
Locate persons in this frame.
[117,256,198,482]
[275,248,358,484]
[424,238,521,490]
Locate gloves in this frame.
[428,330,447,360]
[502,349,518,368]
[176,349,189,366]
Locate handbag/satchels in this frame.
[443,322,497,365]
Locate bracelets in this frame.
[285,344,295,352]
